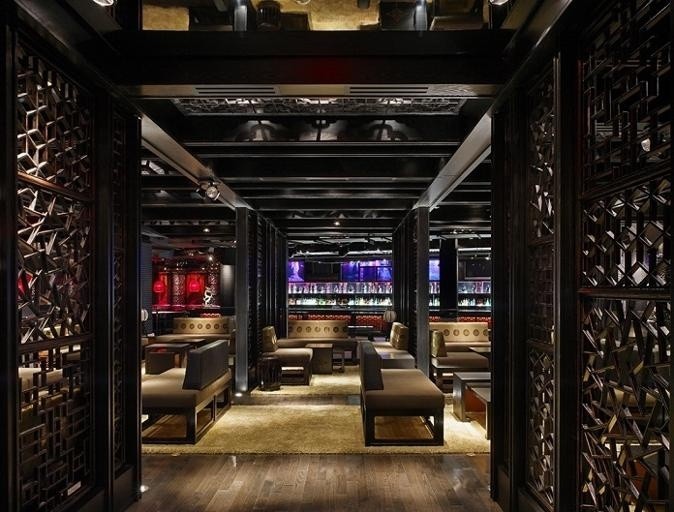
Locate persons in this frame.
[287,261,304,282]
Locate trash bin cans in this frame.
[257,354,281,391]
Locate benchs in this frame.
[140,316,234,444]
[257,318,493,441]
